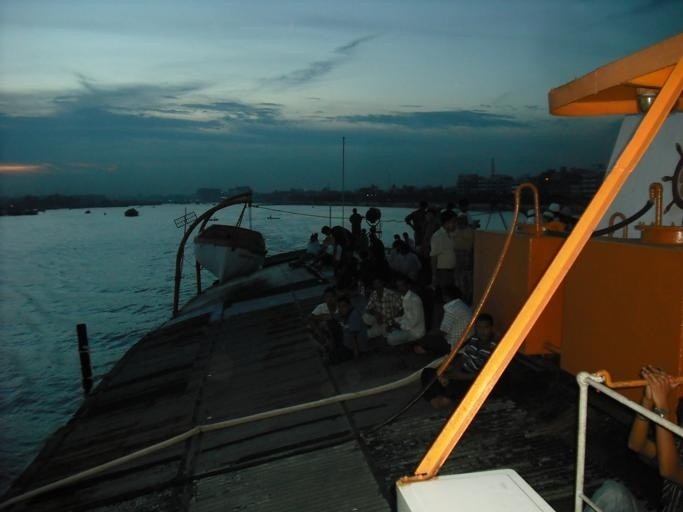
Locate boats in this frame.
[192,223,269,285]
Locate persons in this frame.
[304,198,477,367]
[575,363,682,512]
[419,312,498,410]
[524,202,573,238]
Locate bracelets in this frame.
[654,406,672,417]
[634,408,649,422]
